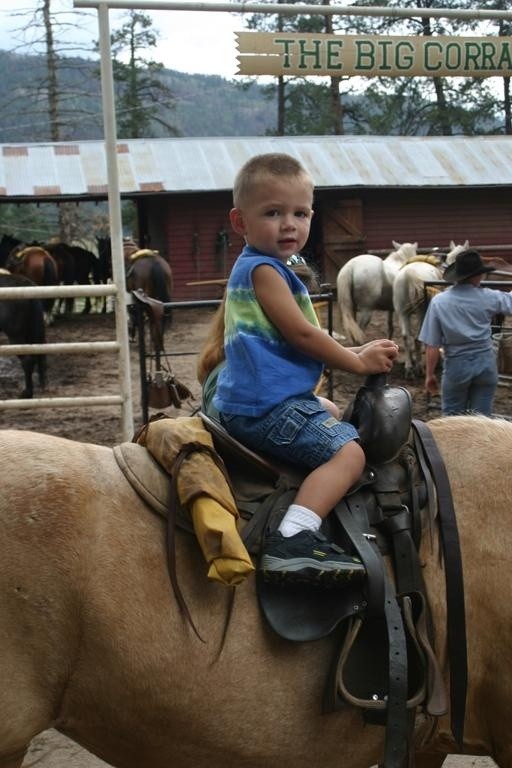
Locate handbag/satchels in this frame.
[148,367,191,409]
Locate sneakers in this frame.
[259,527,366,592]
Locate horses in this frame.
[0,410,512,768]
[392,240,470,381]
[480,256,512,374]
[95,234,172,352]
[32,240,103,315]
[0,269,49,410]
[0,234,59,327]
[337,240,418,347]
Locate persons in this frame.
[212,154,401,593]
[415,252,512,420]
[196,261,325,411]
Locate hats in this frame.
[442,250,497,282]
